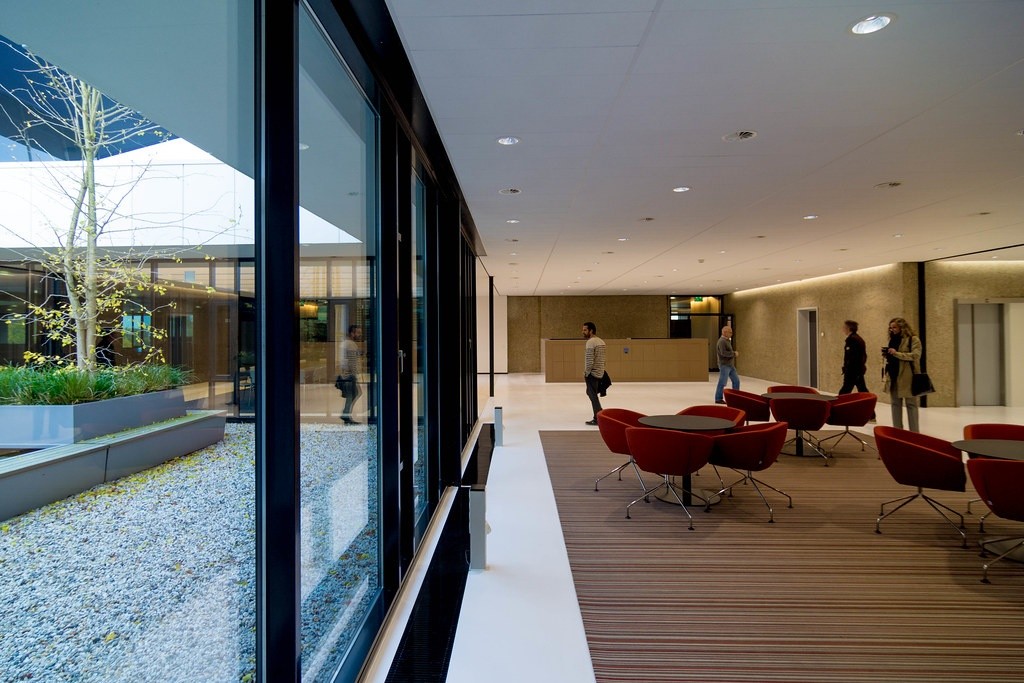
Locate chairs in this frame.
[964,423,1024,532]
[592,408,667,503]
[817,391,878,456]
[677,405,747,496]
[766,385,821,445]
[769,398,834,466]
[966,459,1024,585]
[723,388,770,422]
[622,427,714,529]
[705,421,796,524]
[873,426,969,550]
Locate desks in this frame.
[763,391,839,457]
[951,438,1024,563]
[638,414,736,507]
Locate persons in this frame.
[839,320,877,425]
[338,325,365,426]
[714,326,740,404]
[882,317,922,433]
[581,322,606,426]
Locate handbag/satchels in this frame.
[912,373,936,397]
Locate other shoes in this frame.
[586,419,598,425]
[340,414,360,424]
[715,399,726,404]
[867,416,877,424]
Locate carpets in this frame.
[539,429,1024,683]
